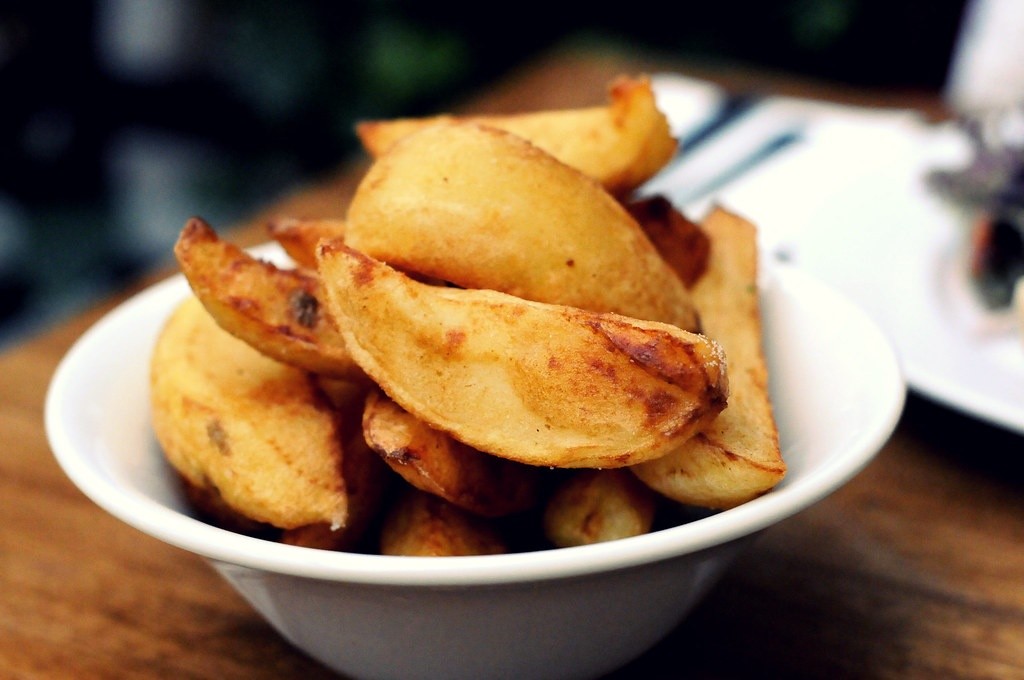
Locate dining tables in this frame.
[0,38,1023,680]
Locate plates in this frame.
[637,96,1024,429]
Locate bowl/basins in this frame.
[46,234,907,680]
